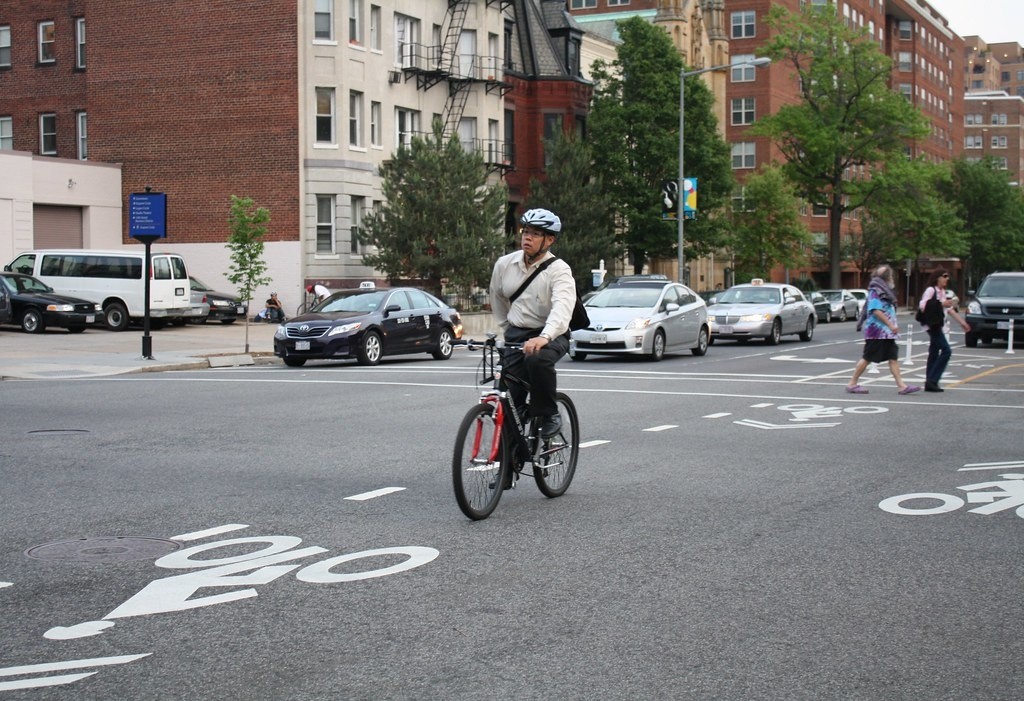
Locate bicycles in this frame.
[296,296,319,317]
[448,331,581,522]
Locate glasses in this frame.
[942,274,948,278]
[519,228,549,237]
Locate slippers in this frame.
[899,385,921,394]
[845,386,869,394]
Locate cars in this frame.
[564,279,711,361]
[170,291,211,326]
[0,273,106,335]
[847,289,869,314]
[706,277,819,344]
[819,289,860,322]
[800,291,831,323]
[186,275,249,324]
[581,274,668,308]
[273,281,463,367]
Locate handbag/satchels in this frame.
[916,287,937,322]
[568,292,590,332]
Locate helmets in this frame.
[271,292,277,296]
[521,208,562,234]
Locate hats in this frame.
[307,285,312,293]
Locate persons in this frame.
[917,269,972,392]
[846,265,921,395]
[265,291,286,323]
[307,284,331,307]
[489,208,576,491]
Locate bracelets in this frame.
[540,333,550,339]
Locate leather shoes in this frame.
[541,413,562,438]
[487,470,519,490]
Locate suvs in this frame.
[3,248,193,332]
[964,270,1024,348]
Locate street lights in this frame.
[676,55,771,283]
